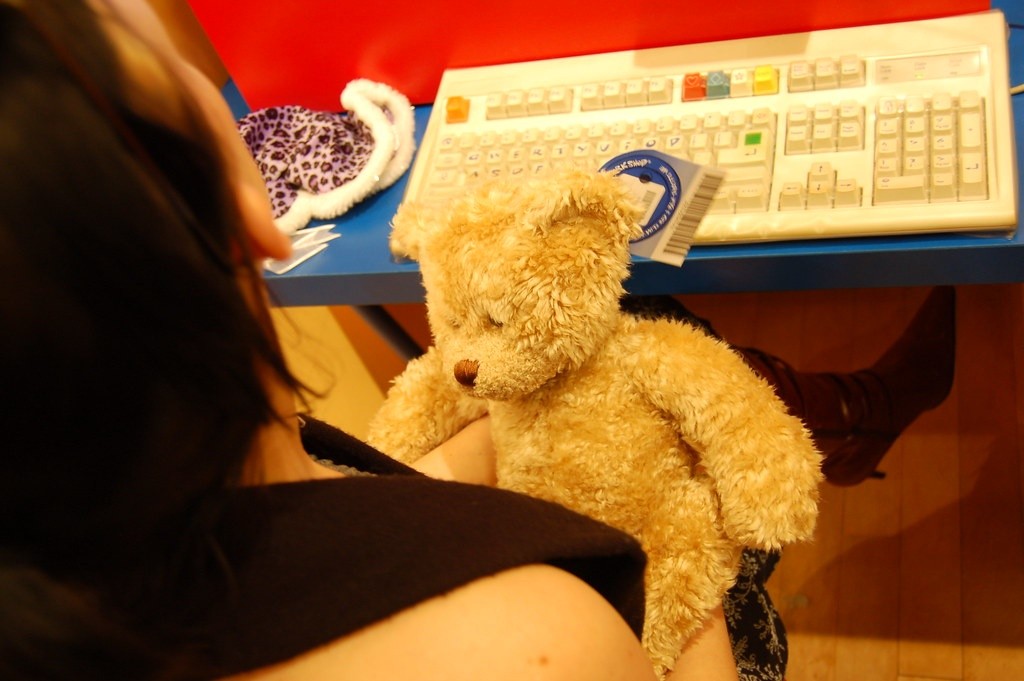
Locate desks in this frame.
[221,0,1023,362]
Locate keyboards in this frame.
[406,8,1018,244]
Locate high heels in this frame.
[820,285,955,487]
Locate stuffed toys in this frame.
[364,172,825,681]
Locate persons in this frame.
[1,0,958,681]
